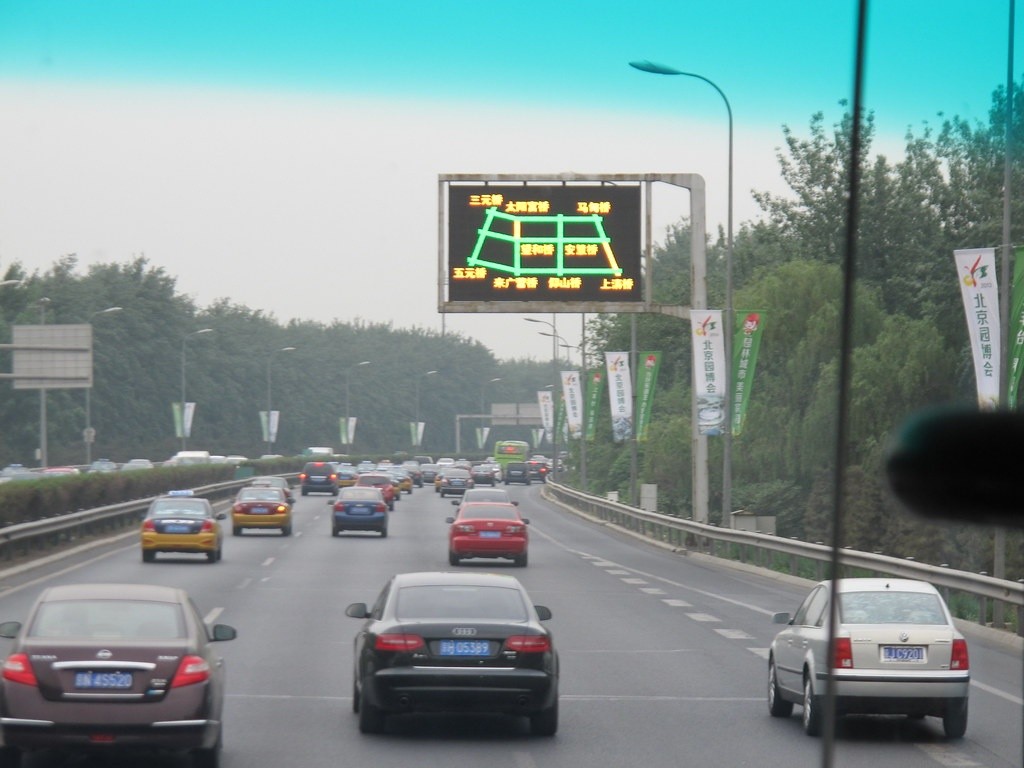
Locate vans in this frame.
[298,461,341,496]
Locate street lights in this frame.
[524,317,588,492]
[181,328,215,452]
[479,377,501,452]
[344,361,372,453]
[84,306,124,464]
[628,59,734,528]
[267,346,296,455]
[414,370,439,453]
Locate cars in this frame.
[334,440,572,511]
[138,490,227,563]
[245,477,296,503]
[345,570,559,739]
[0,446,335,484]
[766,575,972,742]
[326,486,390,537]
[0,582,238,768]
[451,488,518,514]
[230,487,297,536]
[445,501,531,568]
